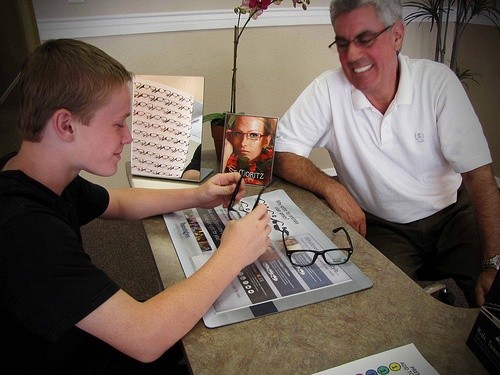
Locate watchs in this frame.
[481,255,500,270]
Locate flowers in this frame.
[202,0,310,131]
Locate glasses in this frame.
[282,226,354,267]
[230,132,269,140]
[329,24,394,53]
[227,175,280,220]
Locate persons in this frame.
[224,115,276,186]
[273,0,500,306]
[0,38,271,375]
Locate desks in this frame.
[125,150,500,375]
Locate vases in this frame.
[212,118,225,162]
